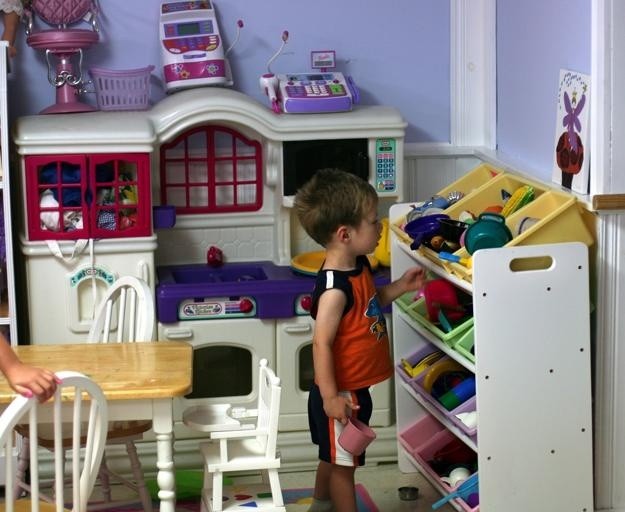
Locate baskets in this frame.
[88,65,155,111]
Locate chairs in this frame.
[182,359,289,509]
[1,279,153,511]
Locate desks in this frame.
[5,344,196,512]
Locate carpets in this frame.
[104,479,382,511]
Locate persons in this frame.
[1,329,63,406]
[294,169,428,512]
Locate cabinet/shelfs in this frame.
[387,201,598,512]
